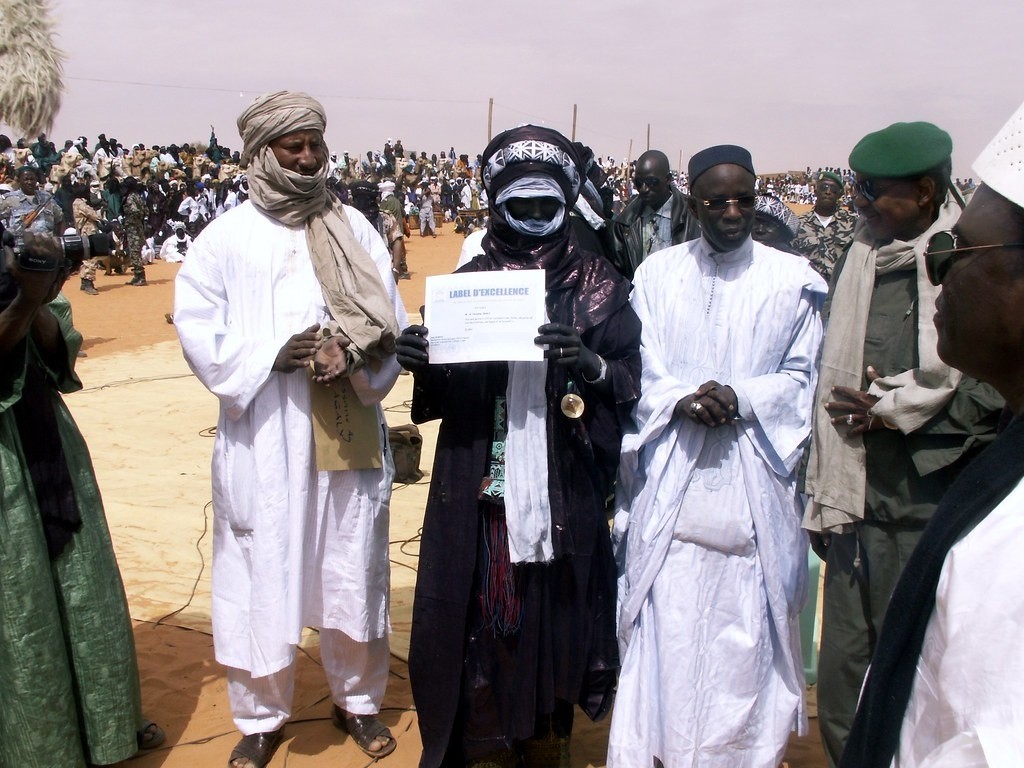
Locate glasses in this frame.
[694,195,756,210]
[922,227,1023,285]
[819,183,840,193]
[851,179,918,203]
[633,176,667,188]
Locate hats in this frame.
[688,145,757,189]
[236,91,398,361]
[479,125,589,259]
[818,171,844,198]
[848,121,953,179]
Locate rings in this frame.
[691,402,703,412]
[847,414,854,426]
[560,348,563,356]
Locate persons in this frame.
[395,124,642,768]
[798,121,1018,768]
[0,130,490,296]
[0,1,167,768]
[606,145,830,768]
[569,142,978,295]
[837,98,1024,768]
[173,90,410,768]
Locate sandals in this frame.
[136,717,165,750]
[228,724,286,768]
[332,704,397,759]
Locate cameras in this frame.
[13,232,112,266]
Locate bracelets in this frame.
[582,354,607,385]
